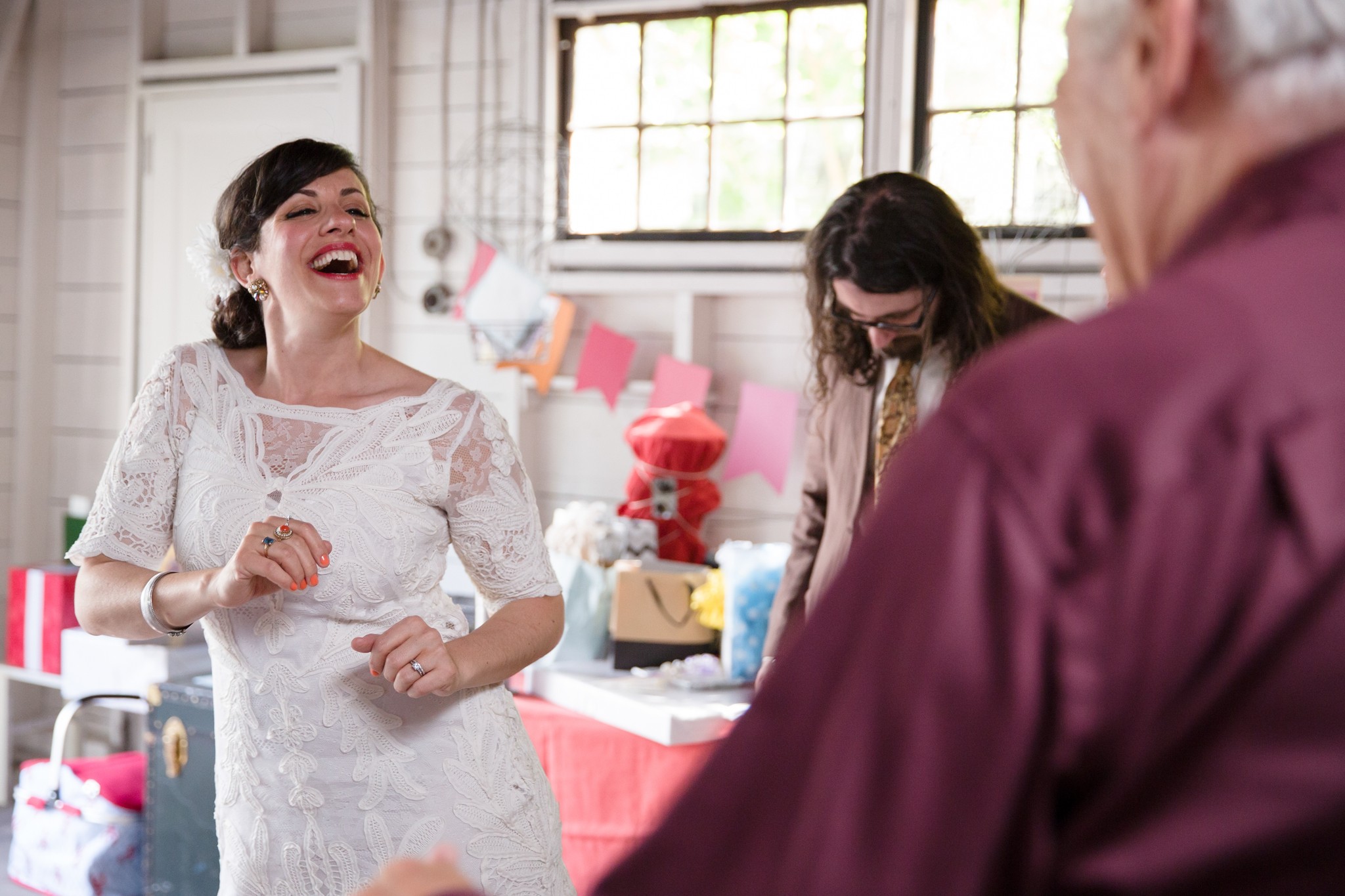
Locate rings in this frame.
[274,525,294,542]
[286,515,291,526]
[262,536,277,559]
[409,660,425,678]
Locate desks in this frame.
[514,694,752,896]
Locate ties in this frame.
[871,362,914,495]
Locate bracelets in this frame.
[140,570,196,638]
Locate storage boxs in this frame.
[6,567,213,715]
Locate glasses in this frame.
[827,284,938,333]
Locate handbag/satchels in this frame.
[610,568,723,671]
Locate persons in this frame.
[361,0,1345,896]
[62,141,580,895]
[754,172,1075,701]
